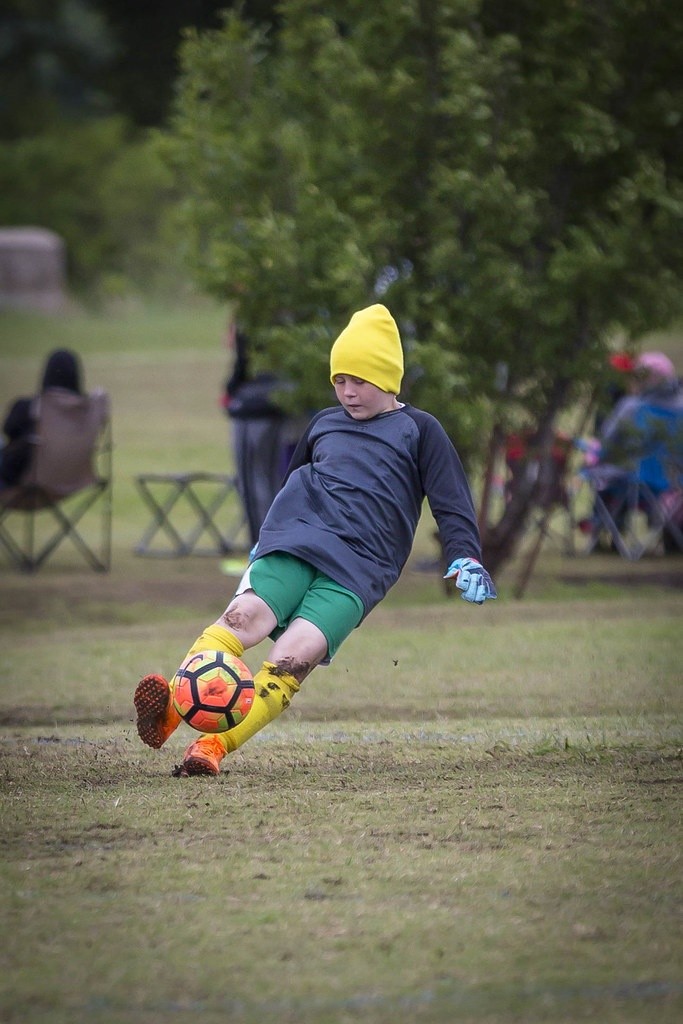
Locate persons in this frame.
[0,350,85,492]
[134,304,497,779]
[573,352,683,553]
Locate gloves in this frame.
[443,558,497,605]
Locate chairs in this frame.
[0,388,114,580]
[561,404,683,564]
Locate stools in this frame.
[129,472,254,558]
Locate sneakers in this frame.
[171,735,228,777]
[134,674,181,750]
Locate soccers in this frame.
[169,650,256,735]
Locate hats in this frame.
[330,304,404,396]
[610,351,674,378]
